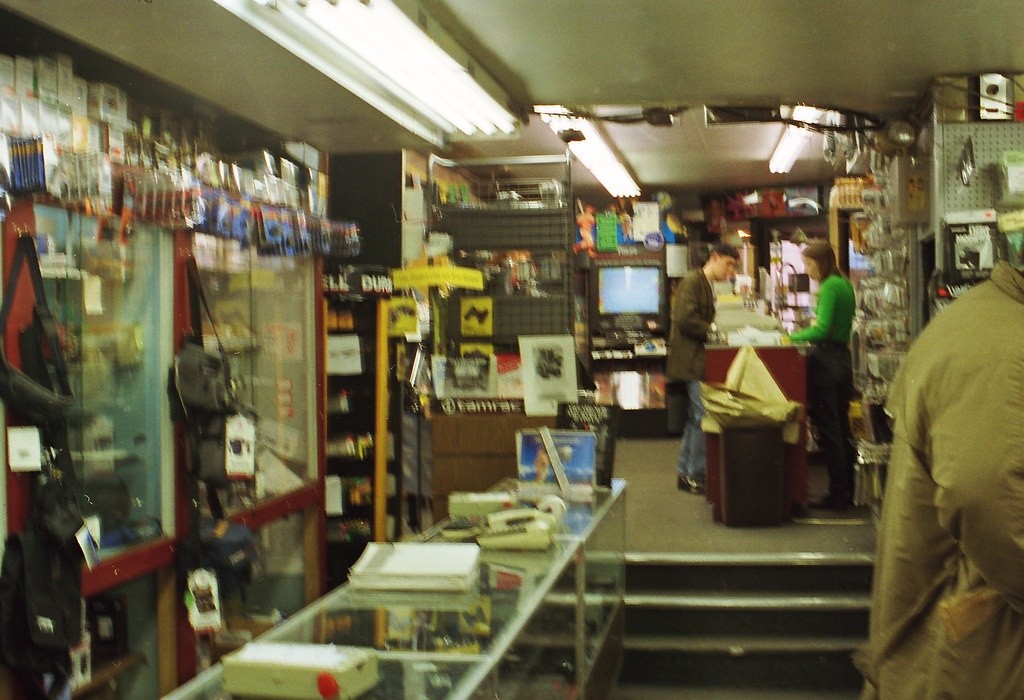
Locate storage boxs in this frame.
[943,208,1005,283]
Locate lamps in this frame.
[533,104,642,197]
[214,0,523,150]
[769,104,824,174]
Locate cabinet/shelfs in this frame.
[0,126,629,700]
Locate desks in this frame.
[702,336,811,516]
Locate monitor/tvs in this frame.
[591,263,666,330]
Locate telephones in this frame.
[475,506,557,554]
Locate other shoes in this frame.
[809,494,852,509]
[678,474,704,494]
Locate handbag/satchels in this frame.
[1,235,131,675]
[165,255,262,594]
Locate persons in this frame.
[665,242,740,495]
[848,240,1024,699]
[779,240,856,510]
